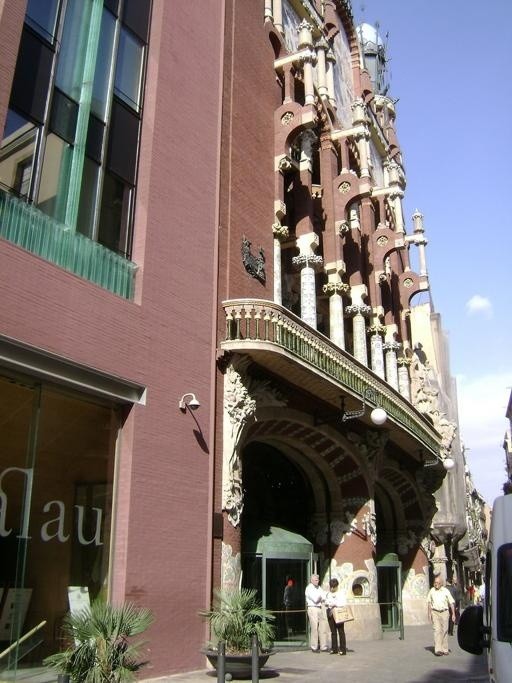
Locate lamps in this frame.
[418,443,456,470]
[179,392,200,409]
[340,395,387,426]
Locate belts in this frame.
[431,608,449,614]
[307,605,322,609]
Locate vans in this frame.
[456,494,512,683]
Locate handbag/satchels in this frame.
[330,604,355,624]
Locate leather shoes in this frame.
[435,651,444,656]
[444,651,449,655]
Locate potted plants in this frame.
[197,583,280,680]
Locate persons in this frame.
[443,577,459,636]
[324,578,347,654]
[303,574,329,652]
[426,575,457,656]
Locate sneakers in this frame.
[337,650,346,656]
[312,649,320,654]
[329,649,338,654]
[321,649,330,652]
